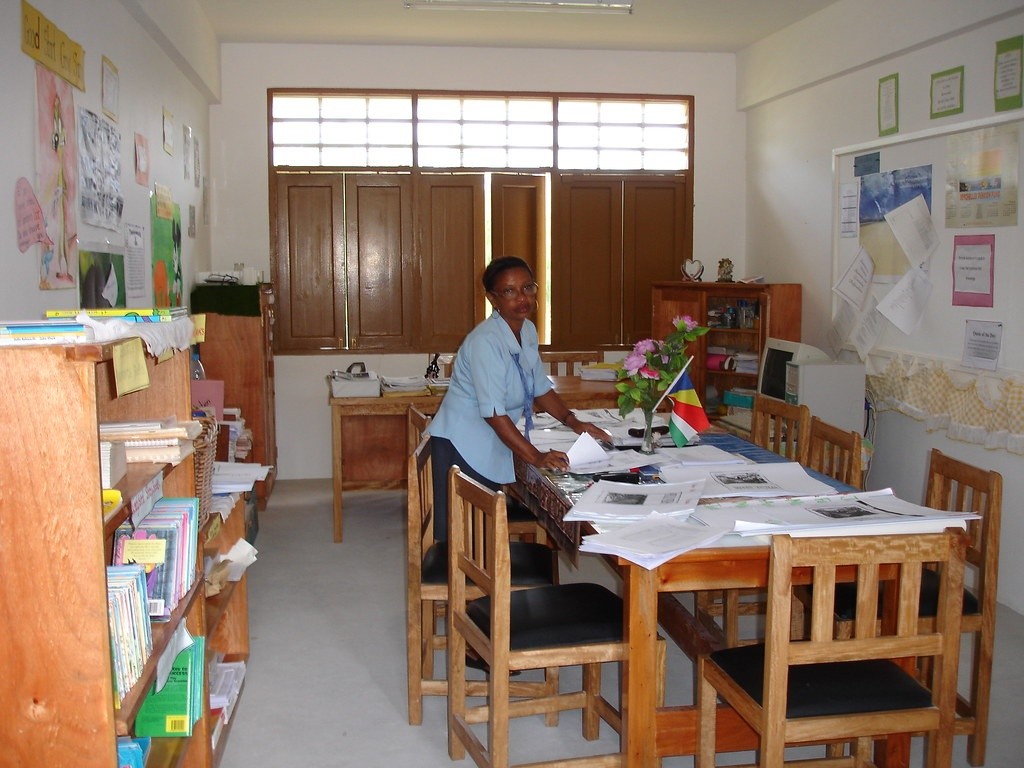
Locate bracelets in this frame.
[563,411,575,427]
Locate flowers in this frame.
[614,314,711,420]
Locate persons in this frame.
[424,255,612,675]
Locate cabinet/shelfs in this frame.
[0,318,249,768]
[190,283,278,512]
[650,281,802,424]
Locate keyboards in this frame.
[721,411,788,438]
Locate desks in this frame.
[329,376,636,542]
[505,412,975,768]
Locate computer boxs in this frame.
[783,361,865,440]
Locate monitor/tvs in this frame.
[759,336,831,422]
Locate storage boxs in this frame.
[724,391,754,408]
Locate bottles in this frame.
[718,300,754,328]
[424,353,440,378]
[190,353,206,417]
[706,354,736,371]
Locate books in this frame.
[0,307,189,346]
[380,376,450,397]
[99,409,262,768]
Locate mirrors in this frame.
[680,258,704,282]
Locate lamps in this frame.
[402,0,633,16]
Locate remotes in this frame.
[629,426,671,437]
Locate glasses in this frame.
[492,281,540,299]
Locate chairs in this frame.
[695,416,862,648]
[407,402,560,727]
[750,393,810,466]
[698,527,968,768]
[789,448,1003,766]
[447,466,666,768]
[443,348,604,376]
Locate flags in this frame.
[664,369,711,448]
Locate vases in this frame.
[639,408,655,455]
